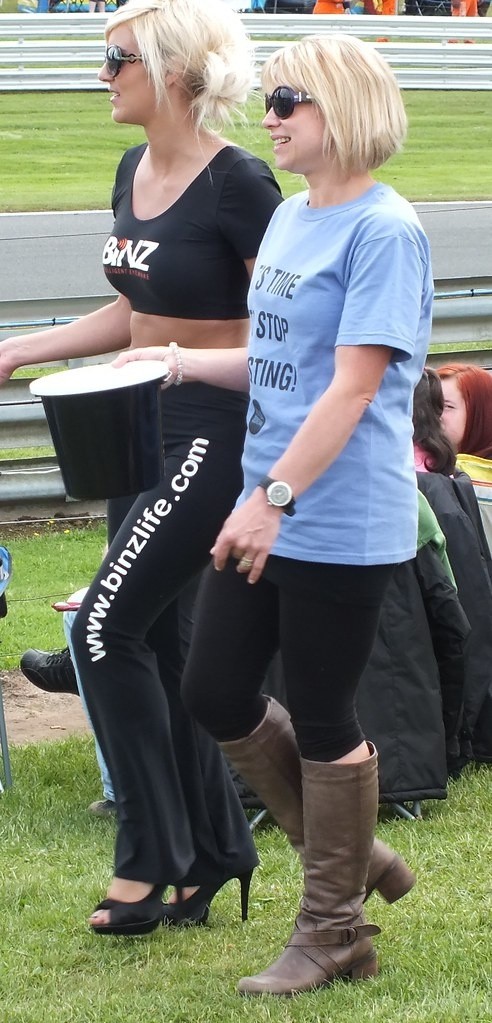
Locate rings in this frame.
[240,556,253,567]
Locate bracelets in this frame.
[170,342,183,385]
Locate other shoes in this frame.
[89,798,119,815]
[230,762,266,808]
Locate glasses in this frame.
[106,46,142,78]
[266,86,312,118]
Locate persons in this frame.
[20,586,127,818]
[1,1,285,933]
[114,33,432,991]
[413,367,492,764]
[224,489,470,807]
[437,364,491,553]
[252,0,492,43]
[90,0,105,12]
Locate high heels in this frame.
[163,868,253,922]
[89,869,184,934]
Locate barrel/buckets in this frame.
[28,359,169,499]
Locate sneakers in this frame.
[20,649,79,697]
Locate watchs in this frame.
[258,475,296,517]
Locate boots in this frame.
[238,741,381,996]
[220,695,414,903]
